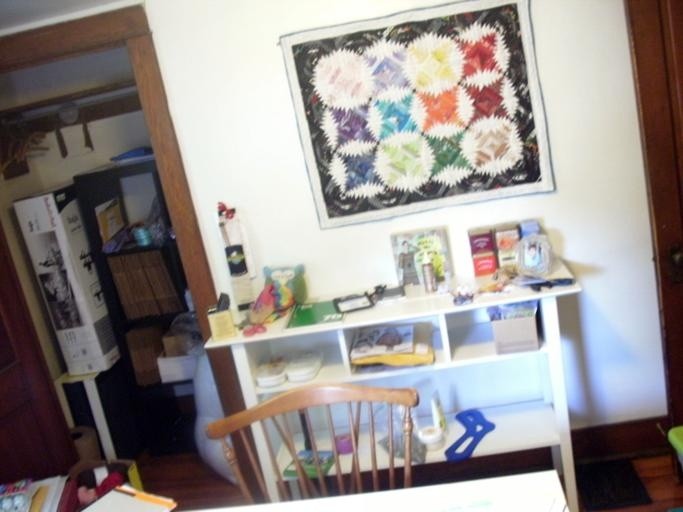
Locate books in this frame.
[352,326,416,358]
[284,447,336,480]
[389,227,455,296]
[93,196,125,244]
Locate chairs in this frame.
[206,385,419,505]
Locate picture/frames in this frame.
[279,0,556,231]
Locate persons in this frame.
[398,239,420,289]
[525,242,542,269]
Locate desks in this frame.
[54,371,119,463]
[174,470,569,512]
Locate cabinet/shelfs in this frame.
[72,156,203,391]
[203,259,581,512]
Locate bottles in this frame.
[420,250,437,293]
